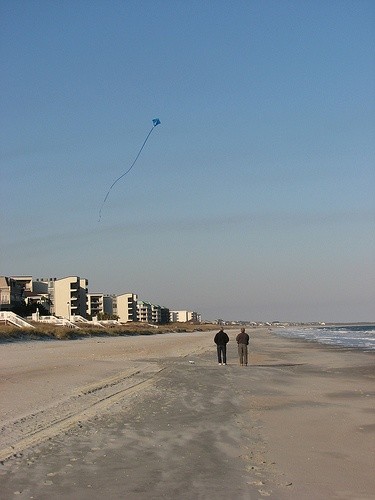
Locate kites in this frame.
[152,117,161,126]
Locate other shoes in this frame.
[240,364,247,366]
[223,363,225,366]
[218,363,221,365]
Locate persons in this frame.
[213,327,230,366]
[235,328,250,366]
